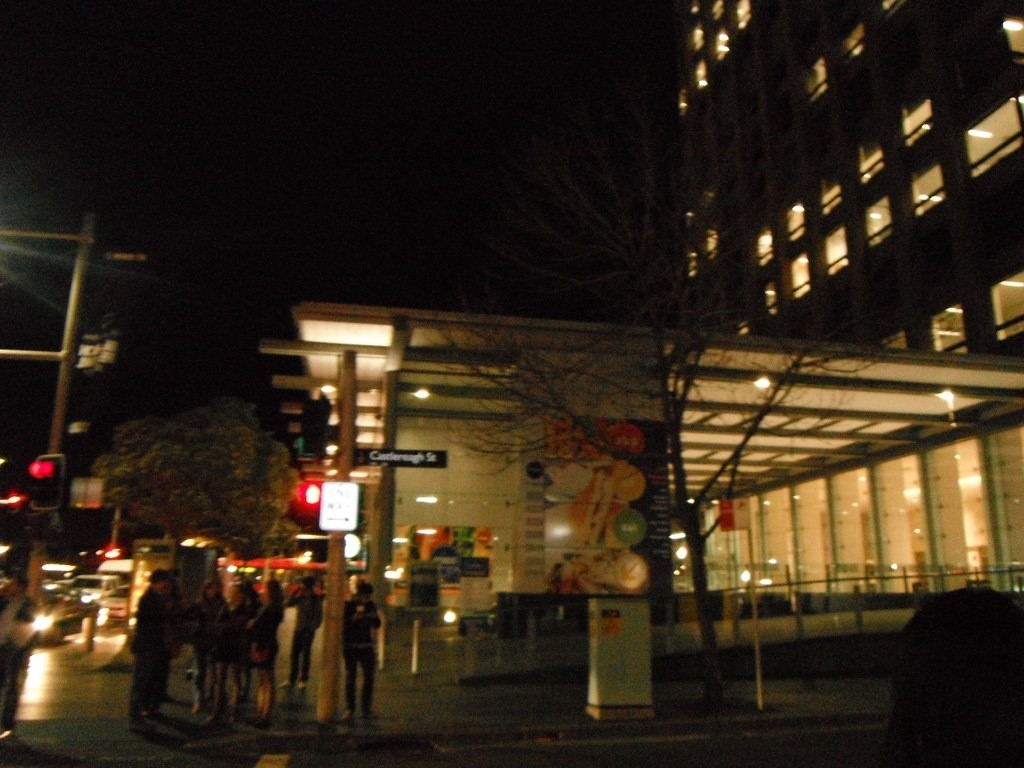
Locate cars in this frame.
[40,559,135,618]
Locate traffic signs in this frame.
[319,482,360,532]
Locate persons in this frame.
[0,573,40,738]
[341,582,384,721]
[126,568,323,728]
[884,587,1024,767]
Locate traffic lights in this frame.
[28,453,67,512]
[297,480,324,520]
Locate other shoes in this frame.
[192,702,205,714]
[363,711,380,718]
[342,708,352,721]
[298,679,307,689]
[281,680,295,686]
[143,709,163,721]
[249,716,271,728]
[129,719,154,733]
